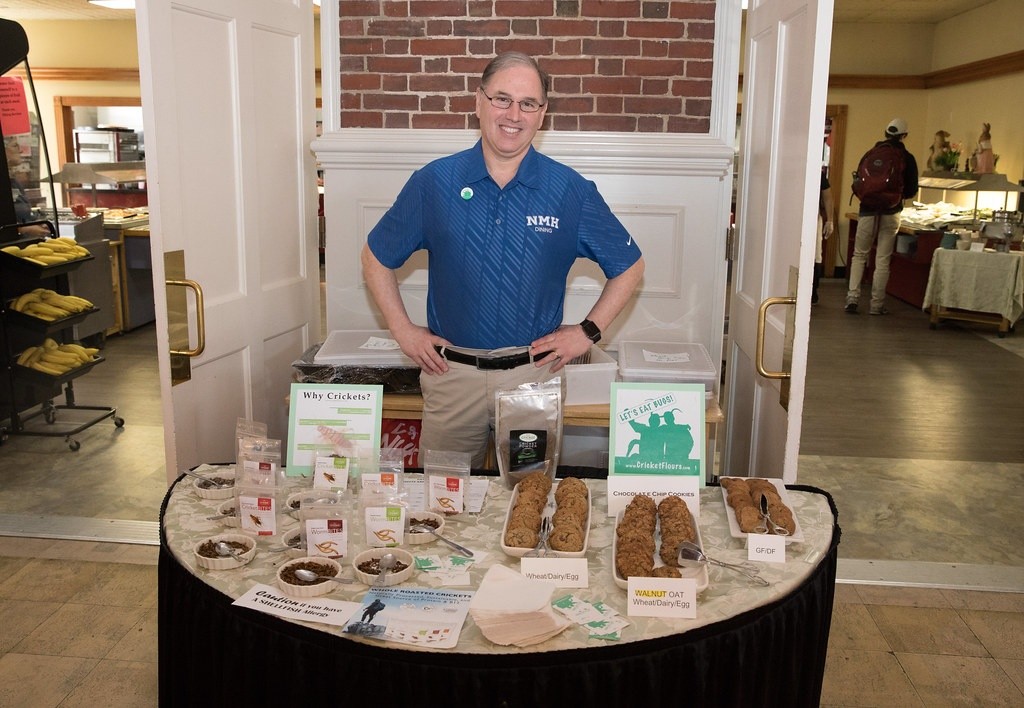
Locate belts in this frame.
[434,345,554,371]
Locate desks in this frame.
[158,466,841,708]
[283,398,726,491]
[922,246,1024,337]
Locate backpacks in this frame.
[849,140,905,217]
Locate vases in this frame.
[943,163,957,172]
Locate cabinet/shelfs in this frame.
[0,221,125,453]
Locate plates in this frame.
[611,508,709,594]
[718,475,806,545]
[500,480,592,558]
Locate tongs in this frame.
[678,540,769,587]
[522,516,559,558]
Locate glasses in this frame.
[480,85,545,113]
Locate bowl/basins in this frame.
[193,472,234,499]
[282,528,307,558]
[193,534,257,570]
[353,547,415,587]
[406,510,445,545]
[217,498,237,527]
[276,556,342,598]
[285,489,341,520]
[956,240,968,250]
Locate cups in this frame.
[944,231,959,249]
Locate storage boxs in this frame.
[291,327,718,470]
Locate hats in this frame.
[885,119,908,136]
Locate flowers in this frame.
[935,140,965,169]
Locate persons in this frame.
[811,169,834,304]
[362,51,644,471]
[844,118,919,315]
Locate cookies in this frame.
[720,477,796,536]
[617,494,697,579]
[504,472,588,552]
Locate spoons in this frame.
[375,553,396,583]
[413,525,474,558]
[215,542,249,563]
[294,569,353,586]
[181,469,231,488]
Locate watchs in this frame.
[580,318,603,343]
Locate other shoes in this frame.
[870,308,889,315]
[812,294,819,306]
[845,301,857,312]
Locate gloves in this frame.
[823,221,833,239]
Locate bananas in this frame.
[16,338,100,375]
[10,288,93,323]
[0,237,91,266]
